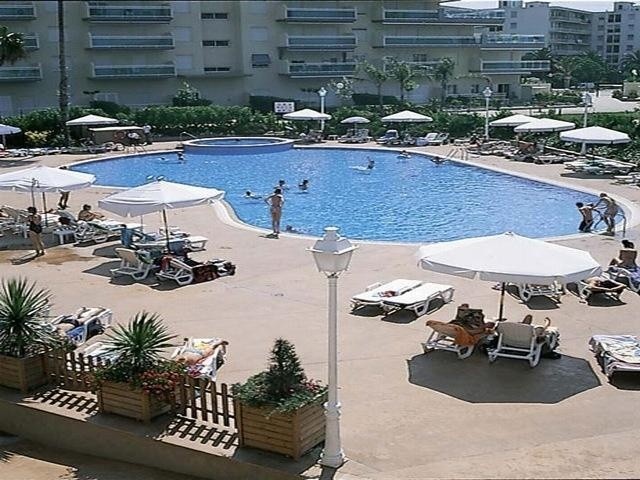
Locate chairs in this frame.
[484,322,558,369]
[351,278,423,313]
[610,257,640,293]
[381,282,453,316]
[589,334,639,388]
[0,128,234,288]
[296,122,640,188]
[509,279,564,303]
[420,309,495,360]
[572,270,627,301]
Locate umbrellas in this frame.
[413,228,604,322]
[560,122,633,166]
[0,123,22,138]
[489,113,539,127]
[340,116,371,135]
[66,114,121,127]
[380,109,434,135]
[514,116,577,134]
[97,175,228,254]
[283,108,332,135]
[0,163,97,226]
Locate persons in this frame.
[576,201,601,232]
[515,142,538,156]
[602,239,635,273]
[522,314,551,337]
[468,135,489,148]
[178,151,186,160]
[264,186,284,234]
[77,203,107,224]
[272,180,287,190]
[53,302,106,338]
[172,252,227,268]
[298,179,310,190]
[56,188,72,212]
[592,192,618,237]
[367,154,375,169]
[26,204,46,256]
[430,156,445,165]
[244,190,254,198]
[127,130,140,147]
[470,134,480,144]
[583,274,625,294]
[400,150,411,159]
[453,302,497,336]
[286,224,299,233]
[142,123,152,145]
[170,335,228,368]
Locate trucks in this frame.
[578,83,596,88]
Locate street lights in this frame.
[482,86,492,140]
[305,226,359,470]
[317,87,328,131]
[580,91,591,153]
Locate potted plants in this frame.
[0,274,73,392]
[88,307,192,421]
[231,337,329,460]
